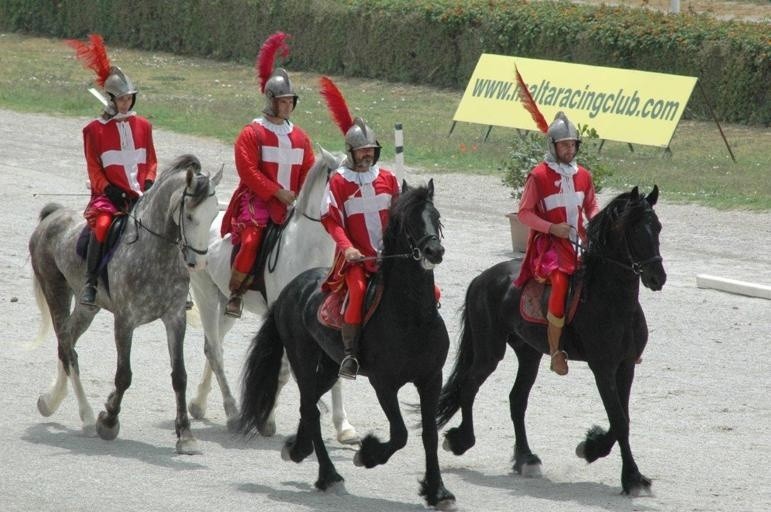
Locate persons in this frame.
[520,112,597,374]
[74,64,156,304]
[320,117,441,380]
[220,64,315,317]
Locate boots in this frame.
[548,312,569,375]
[80,233,105,304]
[228,264,254,316]
[339,323,361,375]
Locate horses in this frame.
[21,153,225,455]
[189,141,364,444]
[233,177,458,511]
[398,185,667,498]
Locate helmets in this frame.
[547,111,581,160]
[102,66,138,115]
[261,68,297,116]
[345,117,383,169]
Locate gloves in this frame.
[106,184,127,207]
[145,179,154,190]
